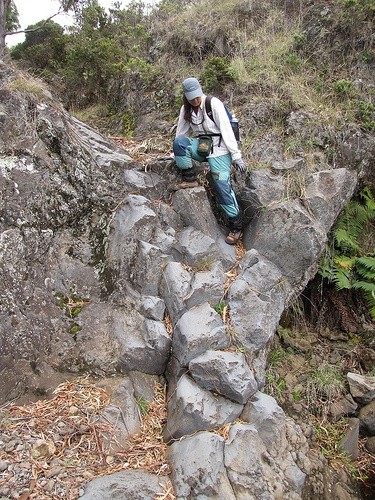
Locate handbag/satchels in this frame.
[198,136,212,157]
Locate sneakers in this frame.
[182,168,195,181]
[226,230,242,245]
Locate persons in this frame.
[173,78,247,245]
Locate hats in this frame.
[182,78,203,100]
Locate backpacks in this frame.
[205,96,239,141]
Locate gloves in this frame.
[234,158,246,172]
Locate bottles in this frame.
[230,114,239,128]
